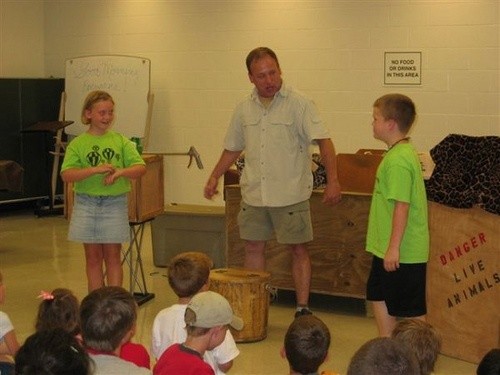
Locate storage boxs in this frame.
[151,204,227,268]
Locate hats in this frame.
[183,291,245,333]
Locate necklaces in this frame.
[387,137,408,151]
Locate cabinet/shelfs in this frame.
[63,155,164,225]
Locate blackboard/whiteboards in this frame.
[63,53,151,139]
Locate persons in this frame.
[153,291,245,375]
[0,273,152,375]
[60,90,148,293]
[151,251,240,375]
[204,47,343,314]
[348,318,441,375]
[365,94,430,338]
[280,315,331,375]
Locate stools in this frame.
[209,268,271,343]
